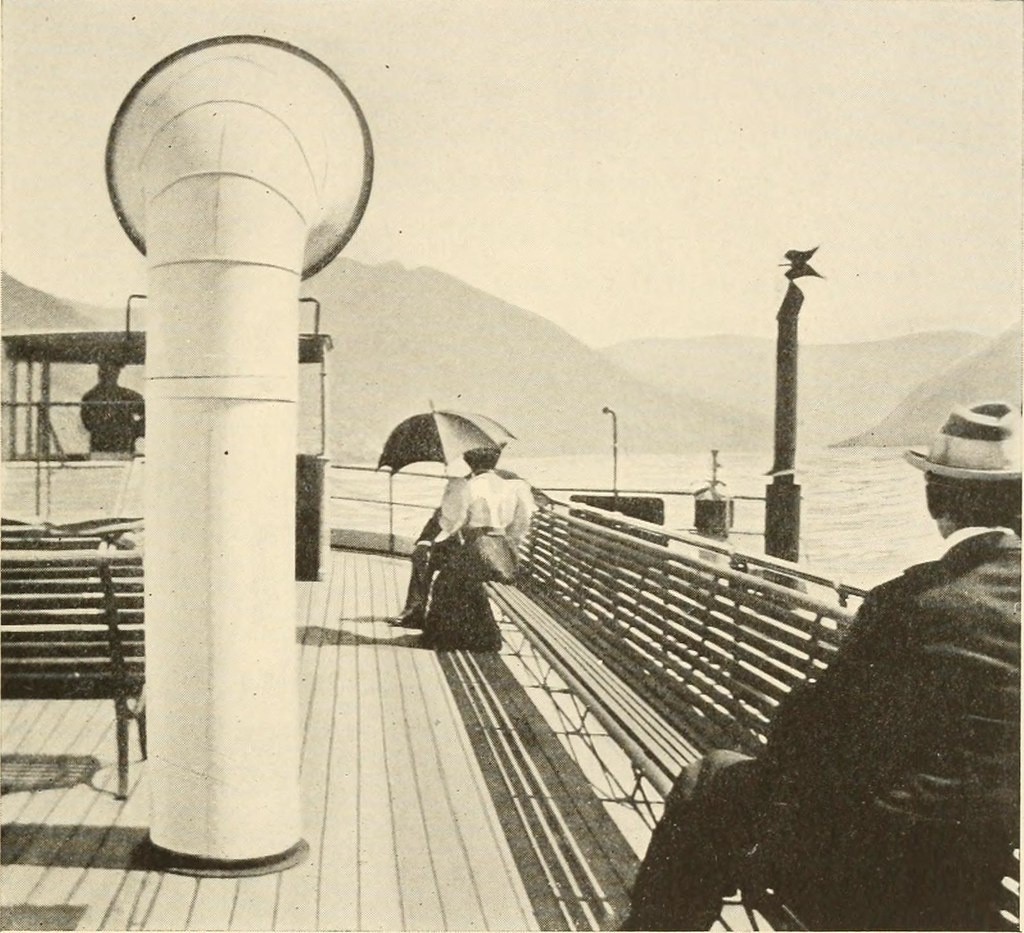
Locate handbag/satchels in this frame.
[459,526,519,584]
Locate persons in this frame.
[81,362,146,462]
[385,448,532,650]
[620,400,1021,932]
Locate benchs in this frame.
[0,555,147,802]
[481,492,1021,933]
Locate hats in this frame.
[903,400,1023,481]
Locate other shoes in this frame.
[388,614,425,630]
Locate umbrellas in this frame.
[374,398,518,476]
